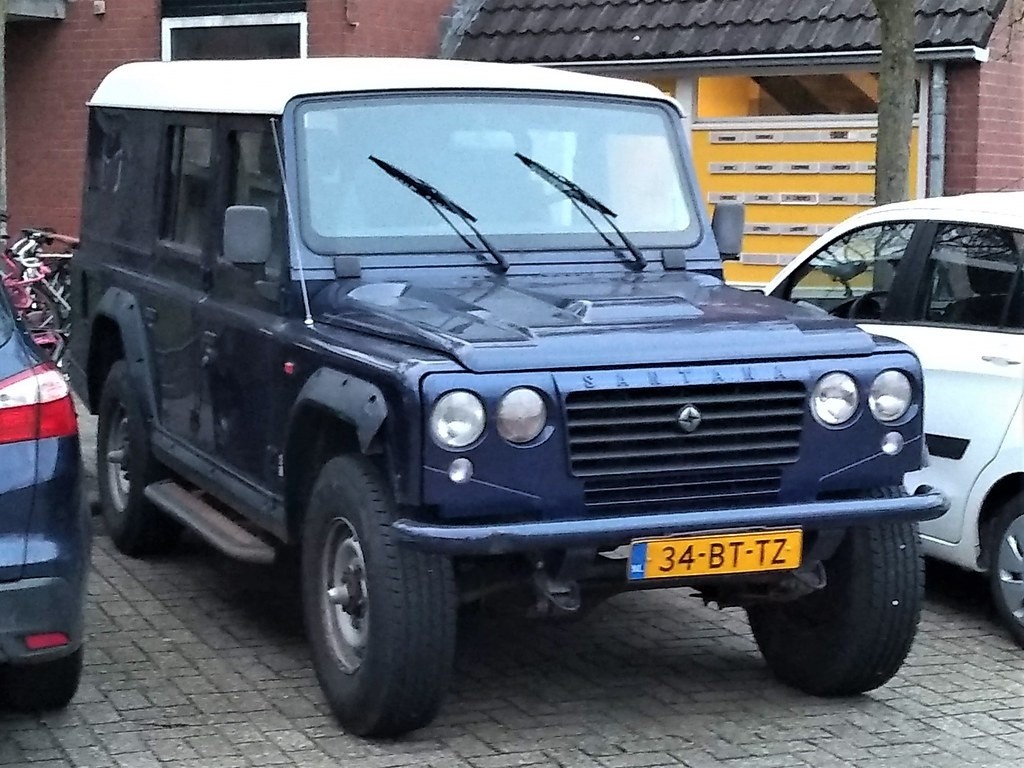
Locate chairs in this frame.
[940,226,1020,326]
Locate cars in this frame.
[0,288,92,710]
[749,188,1024,647]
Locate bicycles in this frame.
[1,209,83,371]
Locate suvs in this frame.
[71,55,949,742]
